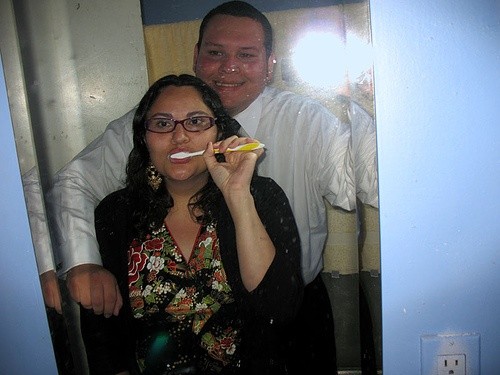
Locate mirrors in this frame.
[0,0,382,373]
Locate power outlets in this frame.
[421,333,480,374]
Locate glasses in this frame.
[142,116,218,134]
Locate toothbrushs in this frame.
[170,143,265,159]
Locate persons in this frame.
[80,74,302,374]
[49,1,356,374]
[20,166,62,319]
